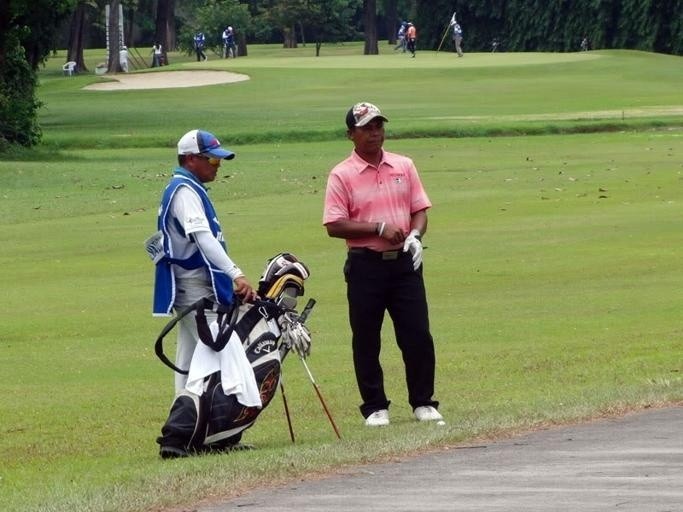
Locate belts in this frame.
[351,248,404,262]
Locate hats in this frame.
[345,103,389,129]
[176,129,235,160]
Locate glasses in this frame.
[192,153,221,166]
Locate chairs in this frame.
[62,61,77,77]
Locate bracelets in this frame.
[378,221,385,237]
[375,223,379,235]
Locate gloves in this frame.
[281,322,312,357]
[403,230,422,272]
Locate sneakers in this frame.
[161,443,254,459]
[414,405,443,422]
[366,409,390,427]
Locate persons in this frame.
[147,129,258,412]
[193,31,208,62]
[322,102,445,426]
[149,40,164,67]
[222,25,236,59]
[394,21,417,58]
[119,46,129,73]
[451,20,463,57]
